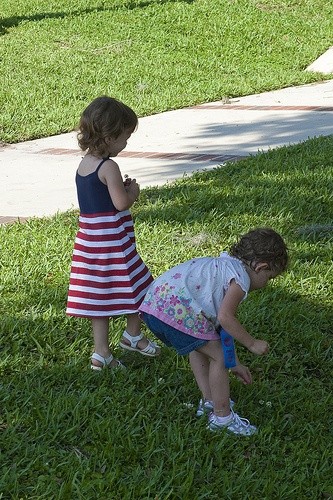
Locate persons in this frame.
[140,228,292,439]
[64,95,162,372]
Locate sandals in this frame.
[120,330,161,357]
[89,353,126,373]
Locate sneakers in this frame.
[204,410,258,437]
[197,399,235,416]
